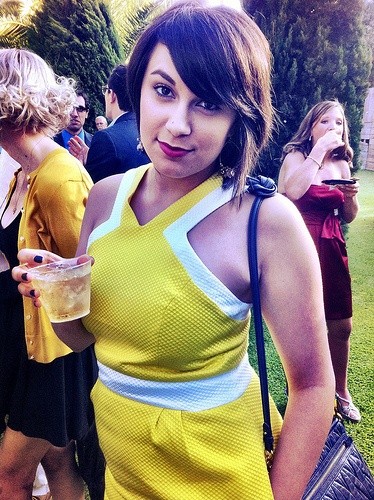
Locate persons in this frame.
[11,0,336,500]
[277,101,362,421]
[95,115,108,131]
[66,64,151,500]
[0,47,95,500]
[50,89,94,151]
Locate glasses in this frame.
[73,106,87,112]
[102,85,109,95]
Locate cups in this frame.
[28,259,90,323]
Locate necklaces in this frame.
[12,135,46,216]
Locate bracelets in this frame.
[307,156,321,167]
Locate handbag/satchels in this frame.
[300,414,374,500]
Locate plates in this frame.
[321,180,356,185]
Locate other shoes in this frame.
[335,391,361,422]
